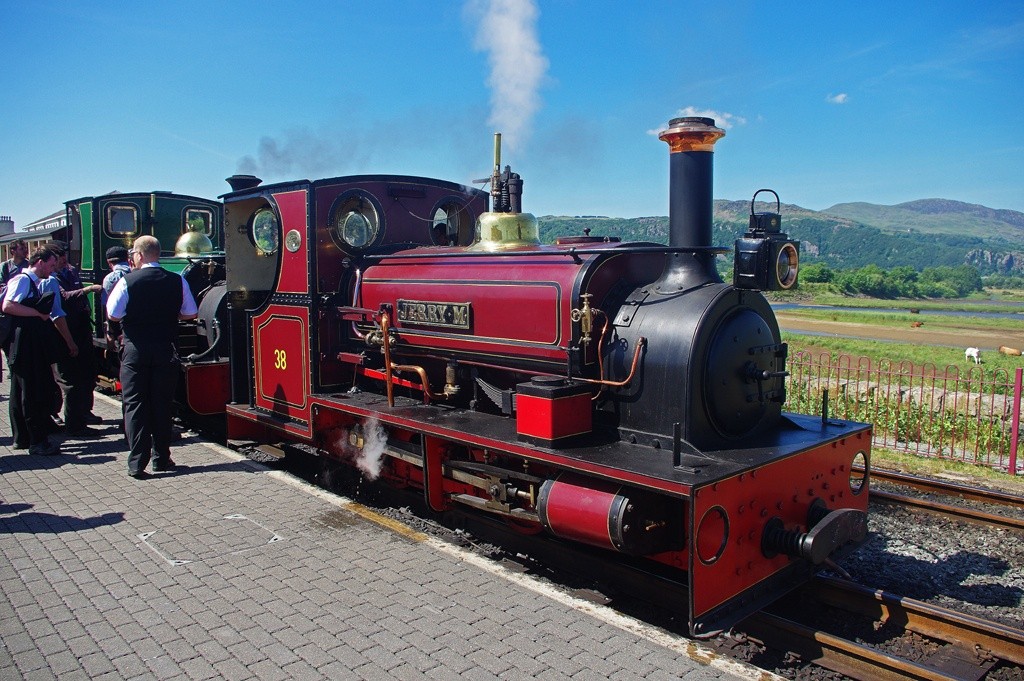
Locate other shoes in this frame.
[50,413,63,424]
[153,458,176,471]
[13,438,31,449]
[85,412,102,424]
[29,443,61,455]
[64,424,99,436]
[128,467,143,477]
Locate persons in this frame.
[0,238,103,456]
[106,234,199,476]
[100,246,131,351]
[127,248,135,271]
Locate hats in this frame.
[47,239,70,252]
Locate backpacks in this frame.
[0,273,34,345]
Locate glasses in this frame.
[128,249,138,257]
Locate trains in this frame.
[0,116,876,645]
